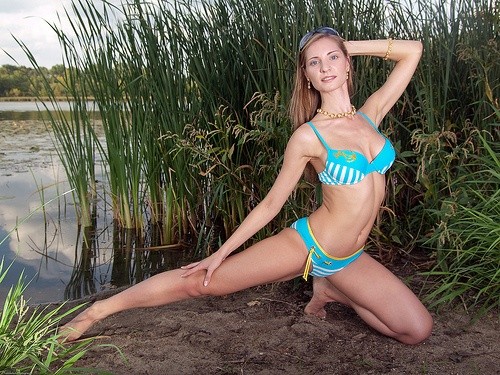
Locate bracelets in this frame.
[383,36,392,61]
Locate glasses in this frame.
[299,26,339,49]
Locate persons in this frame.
[56,26,434,346]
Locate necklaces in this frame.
[317,104,356,118]
